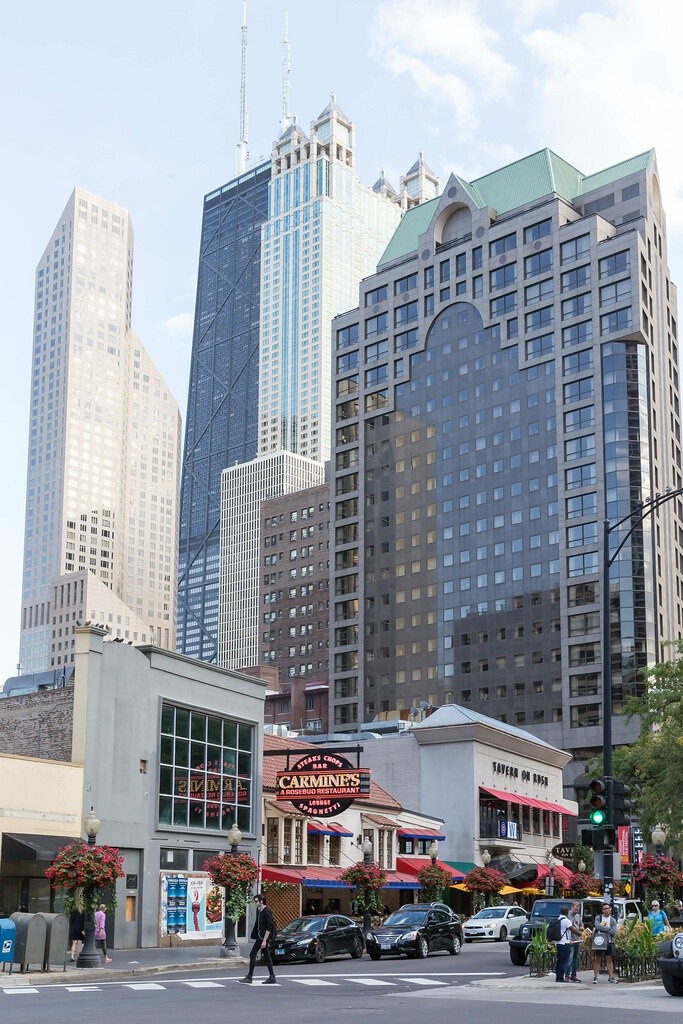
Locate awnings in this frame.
[398,827,446,841]
[478,786,577,816]
[263,858,501,889]
[308,822,353,837]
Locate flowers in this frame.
[537,873,562,887]
[463,866,506,909]
[340,861,388,911]
[44,840,126,917]
[203,854,260,923]
[417,864,452,902]
[632,853,683,909]
[569,873,602,898]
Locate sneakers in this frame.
[262,975,275,984]
[608,978,618,984]
[592,978,598,984]
[105,958,112,963]
[238,975,252,983]
[570,977,581,983]
[565,978,574,983]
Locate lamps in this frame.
[420,701,428,721]
[411,706,419,721]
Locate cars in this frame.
[271,915,366,965]
[656,933,683,997]
[462,906,529,943]
[587,895,650,922]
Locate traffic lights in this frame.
[610,780,632,826]
[590,779,610,828]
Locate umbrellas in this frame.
[449,882,546,912]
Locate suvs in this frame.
[508,899,604,966]
[366,902,463,959]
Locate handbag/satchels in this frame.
[270,922,277,939]
[591,931,607,949]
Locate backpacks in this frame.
[546,917,567,940]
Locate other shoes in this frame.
[555,979,566,982]
[70,958,75,962]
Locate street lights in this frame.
[76,806,102,969]
[219,822,242,957]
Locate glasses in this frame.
[255,900,258,902]
[651,905,657,907]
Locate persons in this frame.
[303,902,391,916]
[555,900,672,985]
[237,894,276,984]
[70,901,113,963]
[501,898,522,907]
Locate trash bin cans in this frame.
[37,912,69,972]
[9,912,47,974]
